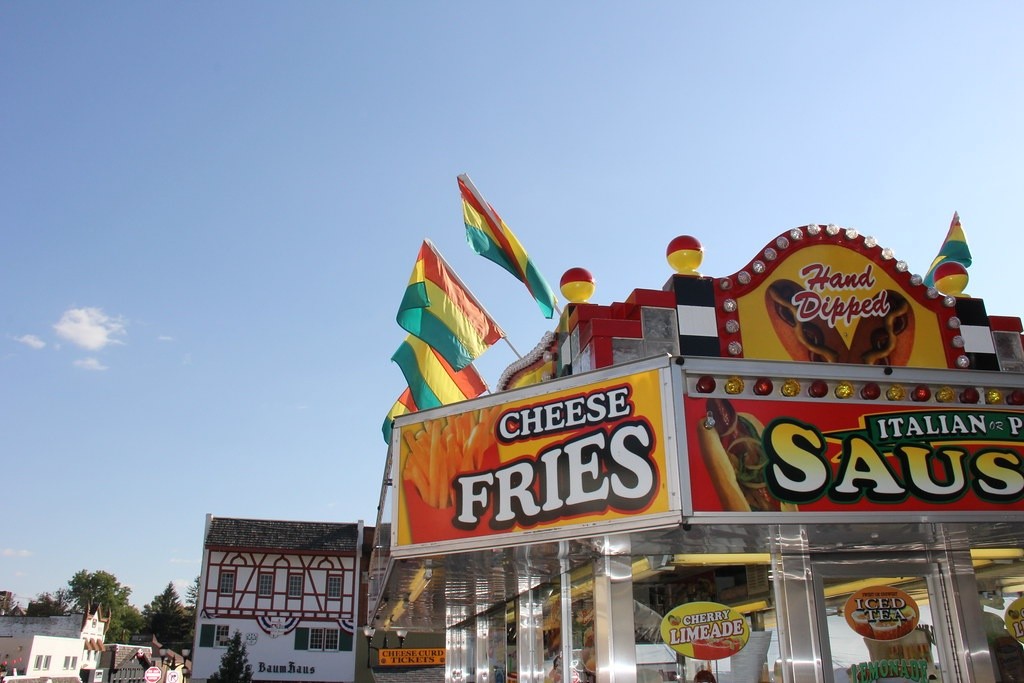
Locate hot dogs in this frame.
[698,399,800,514]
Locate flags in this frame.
[391,334,488,411]
[396,237,507,373]
[922,210,972,286]
[382,387,419,445]
[456,173,559,318]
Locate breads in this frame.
[764,279,850,364]
[851,290,915,368]
